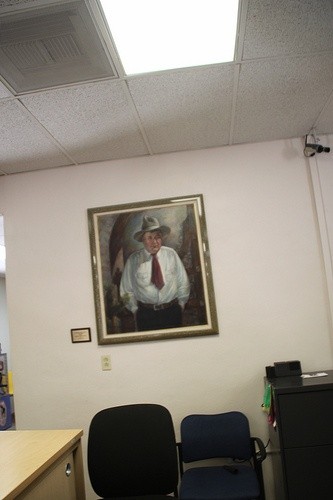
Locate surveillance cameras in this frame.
[305,144,330,154]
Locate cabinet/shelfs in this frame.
[265,370,333,500]
[0,428,86,500]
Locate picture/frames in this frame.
[87,193,219,346]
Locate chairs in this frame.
[175,411,266,500]
[87,404,179,500]
